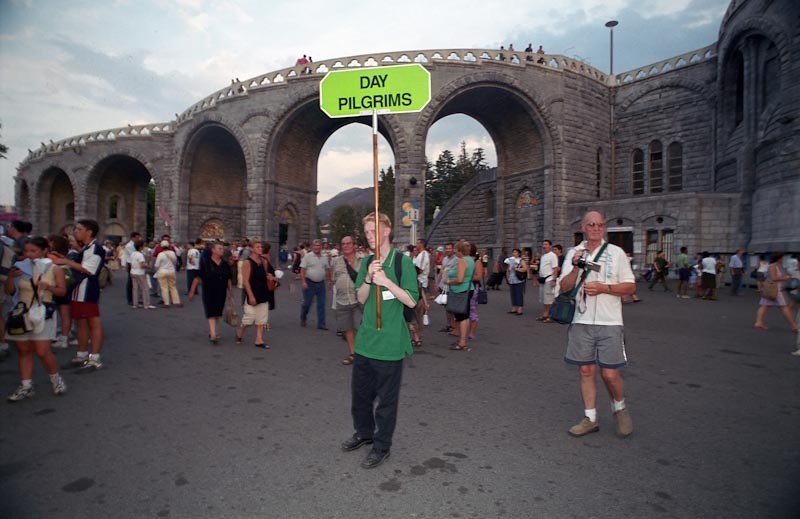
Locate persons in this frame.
[342,213,417,469]
[0,220,800,402]
[563,212,637,436]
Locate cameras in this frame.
[577,256,586,269]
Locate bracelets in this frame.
[608,285,612,294]
[364,279,371,285]
[44,285,50,290]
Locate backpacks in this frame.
[50,251,77,287]
[366,251,414,322]
[99,263,114,289]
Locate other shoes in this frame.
[792,350,800,356]
[487,283,557,323]
[0,324,103,399]
[754,324,768,331]
[612,398,633,437]
[620,287,745,304]
[407,322,474,351]
[209,320,355,366]
[128,292,199,310]
[341,436,374,451]
[568,415,600,437]
[361,445,390,469]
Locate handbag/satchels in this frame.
[515,259,545,284]
[759,280,778,299]
[423,290,469,325]
[224,295,240,327]
[548,286,577,325]
[261,256,275,290]
[5,301,32,336]
[477,288,488,304]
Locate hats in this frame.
[29,304,47,335]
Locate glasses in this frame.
[587,223,606,228]
